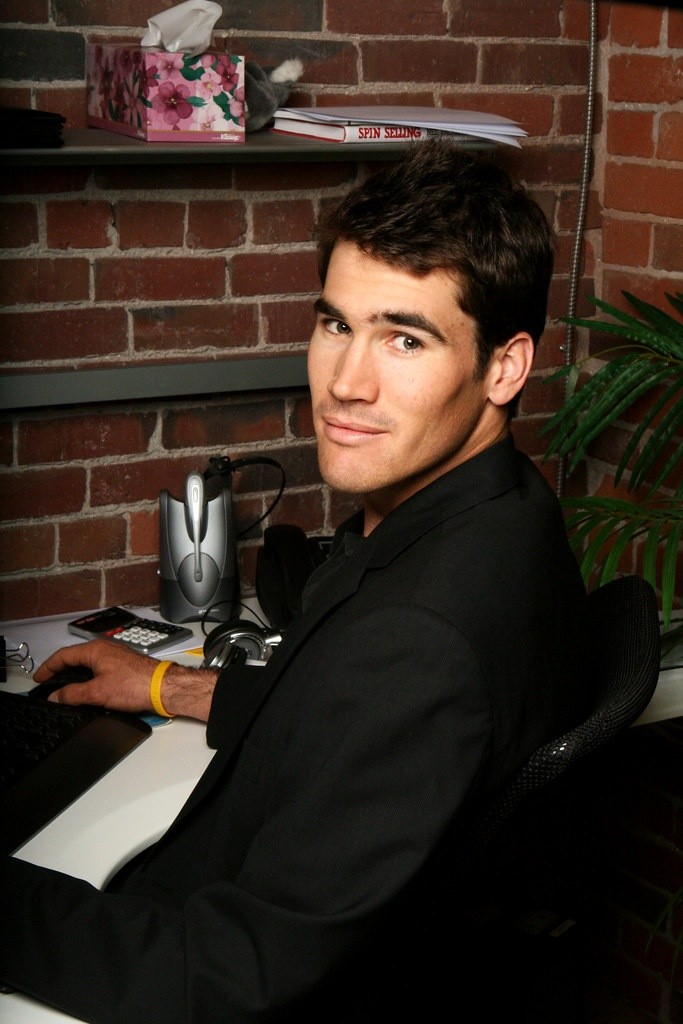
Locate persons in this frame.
[33,142,585,1024]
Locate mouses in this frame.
[27,664,93,699]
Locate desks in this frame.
[0,604,683,1024]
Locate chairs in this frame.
[269,576,656,1024]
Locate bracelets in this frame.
[151,660,176,718]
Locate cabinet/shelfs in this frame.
[1,141,498,412]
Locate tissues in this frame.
[86,0,245,143]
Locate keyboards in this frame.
[0,691,153,858]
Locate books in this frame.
[273,118,430,143]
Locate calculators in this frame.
[67,606,194,655]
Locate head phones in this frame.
[199,619,282,669]
